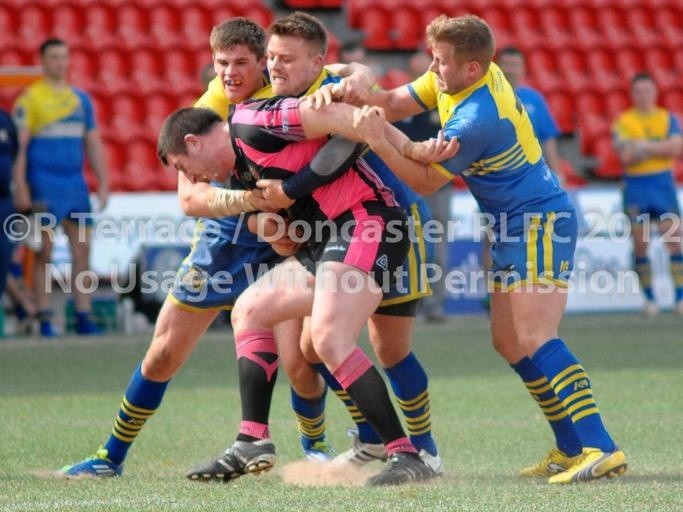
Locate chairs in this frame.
[0,2,680,189]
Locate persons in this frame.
[55,16,378,481]
[400,52,451,324]
[479,46,560,310]
[230,10,443,480]
[296,14,630,487]
[338,42,372,71]
[156,93,463,490]
[0,106,37,340]
[610,73,683,318]
[10,38,110,340]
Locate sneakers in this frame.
[548,446,628,486]
[10,301,106,337]
[301,430,446,489]
[426,305,448,323]
[185,436,276,482]
[519,448,583,479]
[674,301,683,314]
[643,299,661,318]
[61,445,124,481]
